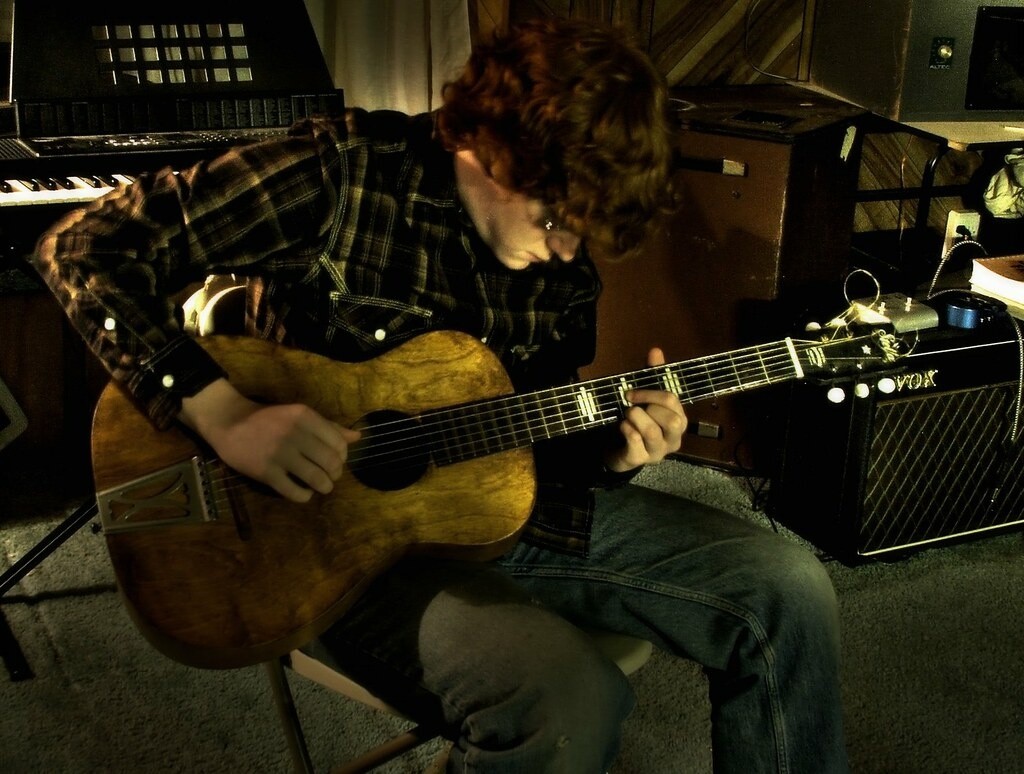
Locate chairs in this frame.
[182,274,652,774]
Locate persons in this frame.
[30,1,847,774]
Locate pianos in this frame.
[0,123,291,240]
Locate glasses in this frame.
[514,155,602,240]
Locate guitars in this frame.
[90,326,898,669]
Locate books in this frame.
[968,254,1024,311]
